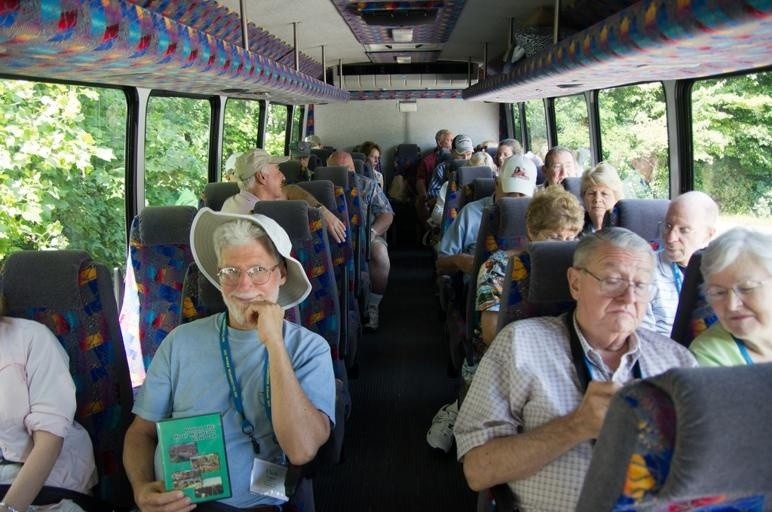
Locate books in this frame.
[156,411,233,504]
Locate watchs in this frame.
[371,228,378,236]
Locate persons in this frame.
[578,165,626,237]
[428,152,495,252]
[494,139,523,174]
[305,136,322,150]
[290,141,315,183]
[0,261,99,512]
[461,188,585,389]
[691,228,772,367]
[435,155,538,322]
[327,149,394,329]
[455,228,703,512]
[416,129,455,230]
[220,147,346,243]
[361,141,384,193]
[641,191,722,339]
[535,147,577,192]
[429,135,474,198]
[123,208,336,511]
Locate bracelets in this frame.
[0,502,19,512]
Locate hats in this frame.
[191,207,312,310]
[289,142,311,157]
[305,135,321,144]
[224,152,244,172]
[235,148,290,181]
[452,135,474,154]
[500,155,537,197]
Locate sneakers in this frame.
[365,304,378,329]
[427,403,453,453]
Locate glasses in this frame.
[575,266,659,302]
[217,264,282,288]
[540,229,577,241]
[658,221,696,236]
[704,279,770,300]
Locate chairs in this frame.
[0,249,134,512]
[130,205,197,402]
[456,197,533,407]
[323,146,335,154]
[437,166,496,310]
[177,263,315,512]
[575,360,772,512]
[279,160,310,185]
[311,164,370,325]
[476,241,576,512]
[444,159,471,182]
[669,246,719,350]
[296,180,361,371]
[435,149,451,168]
[308,153,323,173]
[351,152,372,165]
[352,145,382,173]
[197,182,240,213]
[311,148,330,167]
[603,198,672,252]
[562,177,583,202]
[393,144,421,195]
[485,148,498,166]
[249,199,350,467]
[353,159,372,260]
[445,177,495,381]
[536,166,545,186]
